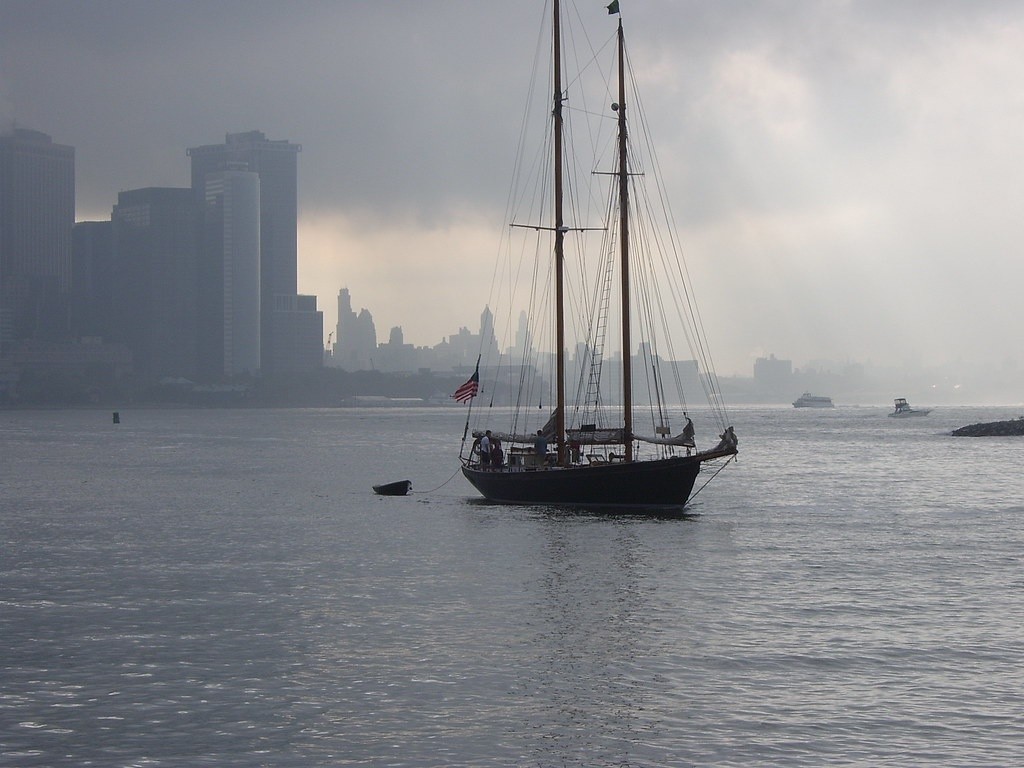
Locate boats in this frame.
[793,390,834,409]
[370,479,414,497]
[888,397,932,418]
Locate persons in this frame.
[481,430,492,470]
[491,443,503,471]
[534,430,547,468]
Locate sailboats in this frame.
[462,0,744,516]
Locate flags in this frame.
[450,370,479,404]
